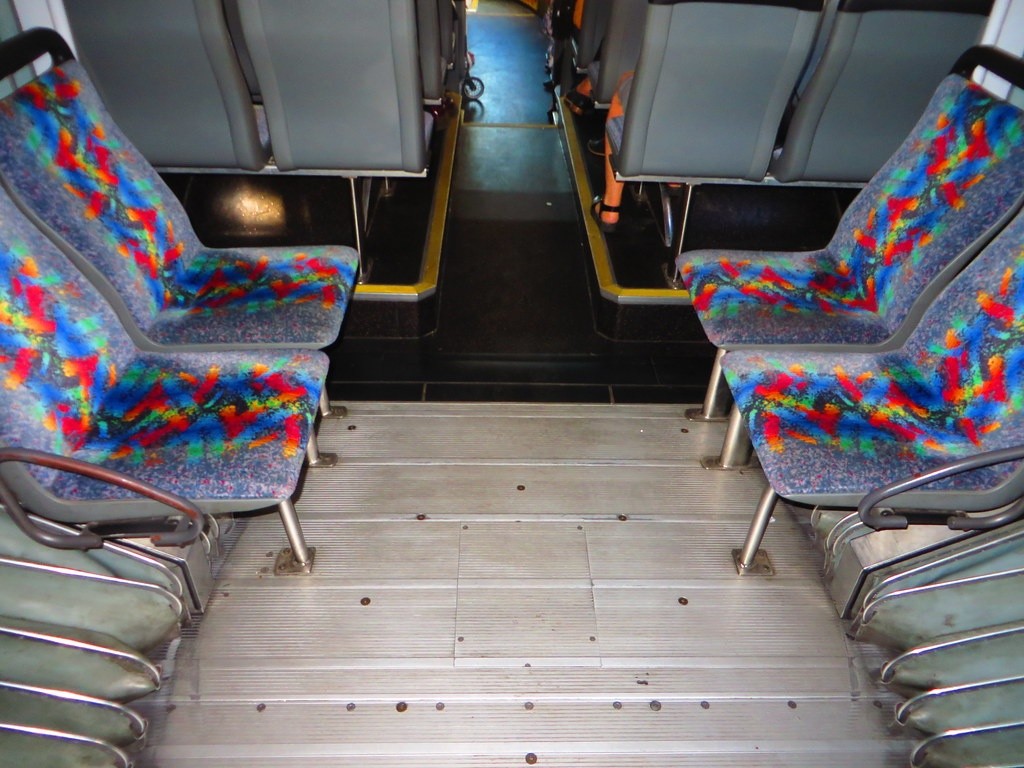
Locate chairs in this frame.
[62,0,998,250]
[0,186,329,580]
[0,27,360,469]
[703,205,1024,580]
[677,44,1024,472]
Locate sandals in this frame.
[591,193,620,232]
[667,182,681,188]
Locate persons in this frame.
[564,34,602,115]
[543,0,584,87]
[589,72,683,233]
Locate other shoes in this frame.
[588,139,605,156]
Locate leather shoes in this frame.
[565,88,590,116]
